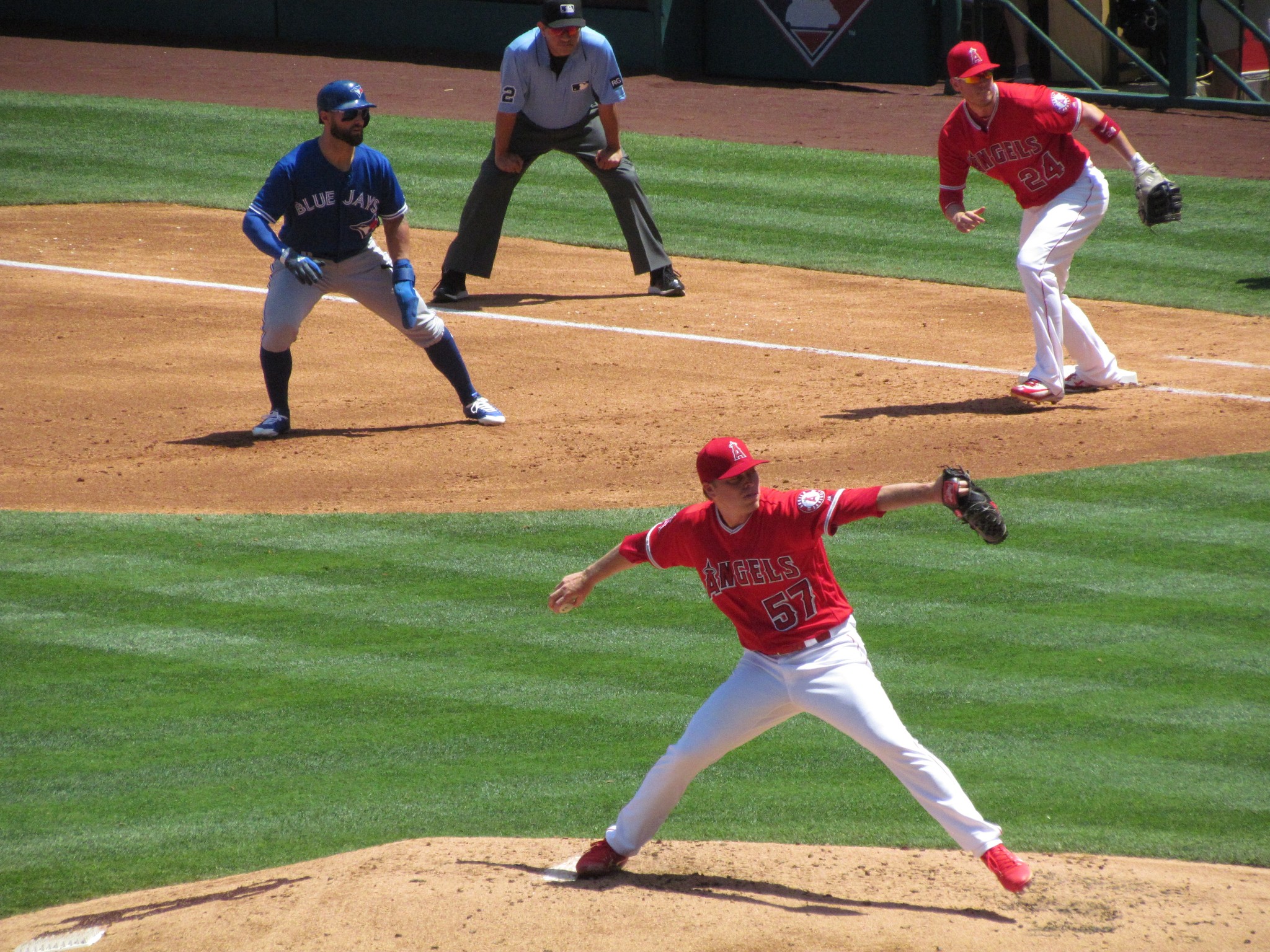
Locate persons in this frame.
[429,0,685,296]
[543,437,1036,890]
[244,80,506,436]
[938,41,1181,403]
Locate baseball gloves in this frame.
[1133,161,1182,228]
[941,467,1006,544]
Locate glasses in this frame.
[959,68,994,84]
[329,109,370,120]
[544,28,581,36]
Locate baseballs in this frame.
[556,600,573,614]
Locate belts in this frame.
[761,629,832,656]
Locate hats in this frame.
[541,0,587,29]
[948,41,1001,79]
[695,436,769,485]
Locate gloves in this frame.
[279,248,326,286]
[390,258,419,329]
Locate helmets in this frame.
[317,79,377,111]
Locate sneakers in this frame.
[1010,379,1064,404]
[461,391,506,426]
[1063,373,1099,393]
[575,838,628,874]
[647,263,685,296]
[251,407,292,439]
[981,844,1032,894]
[430,267,469,301]
